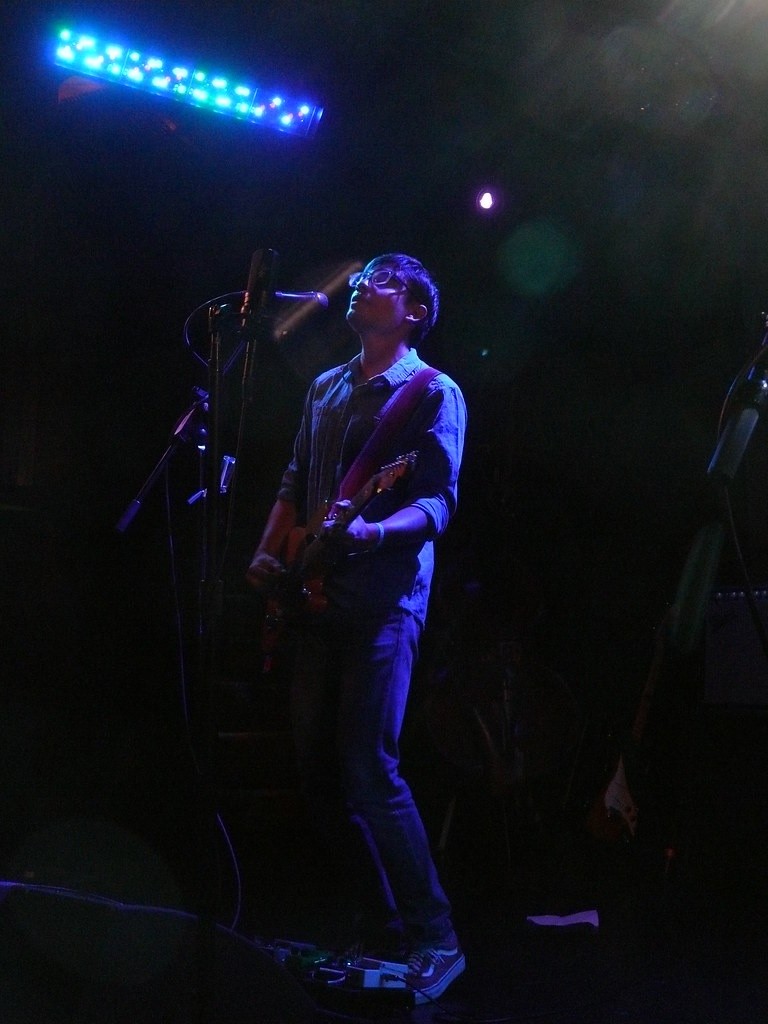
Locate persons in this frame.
[245,254,467,1006]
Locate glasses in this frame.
[349,269,423,304]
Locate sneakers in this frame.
[405,945,466,1004]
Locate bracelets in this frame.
[369,521,385,550]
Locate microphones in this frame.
[270,287,328,313]
[240,247,280,315]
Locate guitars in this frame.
[264,454,418,628]
[568,600,681,859]
